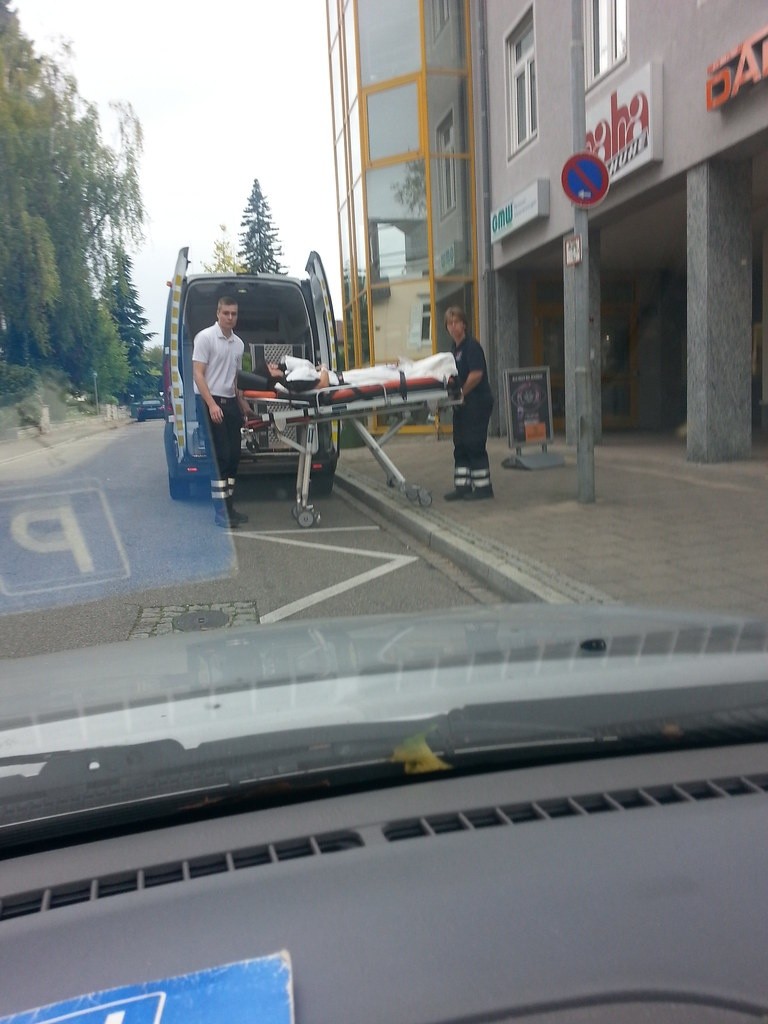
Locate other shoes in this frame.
[215,510,249,528]
[444,487,494,501]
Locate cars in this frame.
[136,398,164,422]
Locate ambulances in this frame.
[163,245,342,501]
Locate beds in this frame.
[237,371,450,529]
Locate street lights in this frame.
[92,372,101,417]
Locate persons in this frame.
[444,306,493,501]
[192,297,248,528]
[253,352,459,392]
